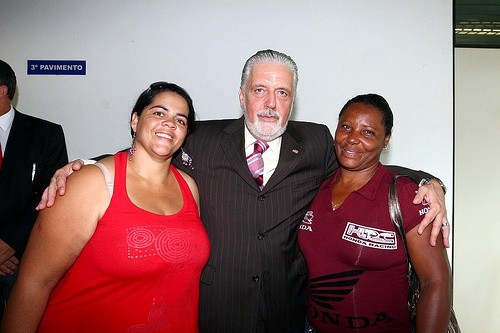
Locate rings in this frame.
[442,222,449,227]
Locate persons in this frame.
[36,49,450,333]
[0,82,212,333]
[0,239,20,276]
[296,92,452,333]
[0,60,69,332]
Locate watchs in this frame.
[423,178,446,196]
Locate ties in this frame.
[246,140,270,187]
[0,143,3,168]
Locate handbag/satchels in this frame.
[408,275,460,333]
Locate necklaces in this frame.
[331,200,344,211]
